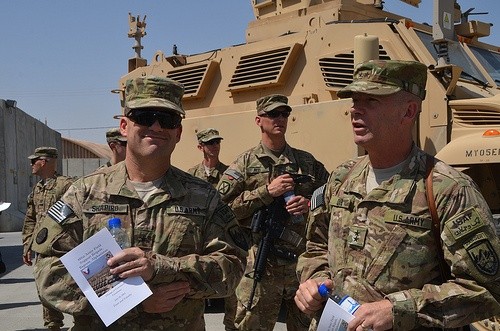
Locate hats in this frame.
[255,94,292,116]
[27,146,59,159]
[106,127,127,144]
[336,60,427,100]
[196,128,224,145]
[111,75,186,116]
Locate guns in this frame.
[244,167,315,311]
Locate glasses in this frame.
[201,139,221,145]
[125,106,183,129]
[117,140,127,146]
[30,158,48,164]
[260,109,290,118]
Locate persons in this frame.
[92,128,128,170]
[22,147,76,331]
[293,60,500,331]
[216,94,329,331]
[32,76,249,331]
[186,129,229,191]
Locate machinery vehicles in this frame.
[104,0,500,239]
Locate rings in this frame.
[361,324,368,331]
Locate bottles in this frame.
[318,283,362,315]
[283,190,304,224]
[109,218,133,263]
